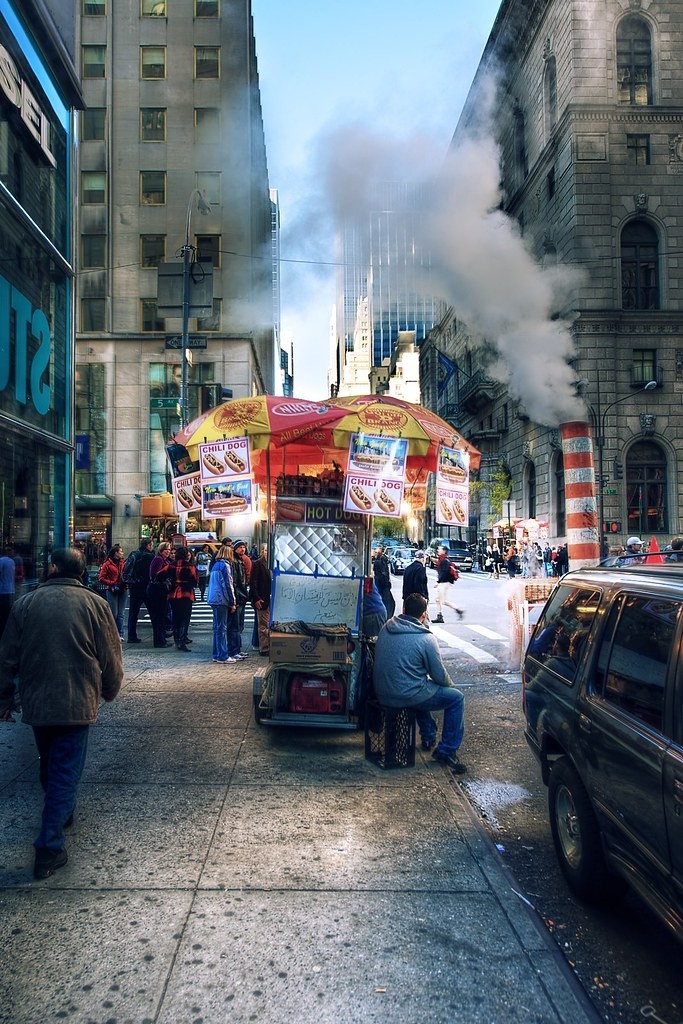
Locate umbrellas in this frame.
[176,393,481,568]
[494,517,548,529]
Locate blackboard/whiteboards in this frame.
[269,571,362,635]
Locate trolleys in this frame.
[252,494,375,731]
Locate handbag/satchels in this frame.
[197,564,209,578]
[110,584,122,593]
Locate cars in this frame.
[370,536,428,575]
[185,538,223,555]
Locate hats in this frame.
[627,537,645,546]
[415,550,428,558]
[233,539,246,550]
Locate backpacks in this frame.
[123,551,144,585]
[445,558,459,581]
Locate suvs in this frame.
[424,537,477,572]
[519,548,683,948]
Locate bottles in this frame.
[276,471,342,496]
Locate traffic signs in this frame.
[164,334,208,350]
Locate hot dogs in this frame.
[353,454,399,471]
[440,498,465,522]
[350,485,396,513]
[178,450,248,515]
[440,465,466,483]
[277,503,305,520]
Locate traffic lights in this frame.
[611,522,621,533]
[602,521,610,533]
[214,385,234,407]
[199,386,214,415]
[612,462,624,481]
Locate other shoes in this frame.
[165,630,173,638]
[177,644,192,652]
[121,637,124,641]
[186,639,193,644]
[252,646,259,650]
[162,643,174,647]
[127,638,141,643]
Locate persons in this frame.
[151,365,212,491]
[612,536,683,564]
[375,545,465,622]
[374,593,467,771]
[98,537,271,664]
[74,367,91,493]
[0,548,122,880]
[485,542,568,579]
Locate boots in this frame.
[457,609,463,618]
[431,614,444,623]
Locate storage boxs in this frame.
[365,703,420,770]
[269,632,347,664]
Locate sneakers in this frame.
[233,654,246,661]
[422,740,436,748]
[213,658,217,662]
[240,651,249,657]
[34,848,67,879]
[432,747,467,773]
[63,821,77,835]
[217,657,236,664]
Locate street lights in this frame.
[575,377,658,560]
[173,187,213,535]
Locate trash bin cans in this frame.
[90,576,107,598]
